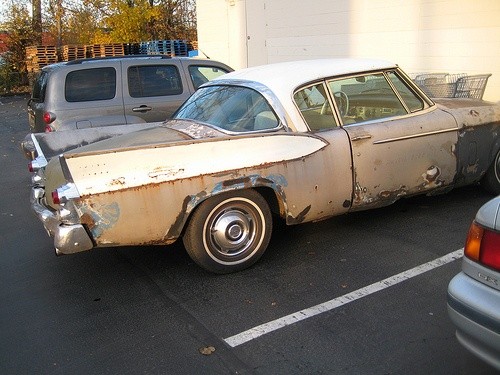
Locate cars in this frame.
[446,194,500,370]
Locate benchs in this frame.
[254,111,356,130]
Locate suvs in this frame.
[27,37,237,132]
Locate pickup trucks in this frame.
[20,58,500,274]
